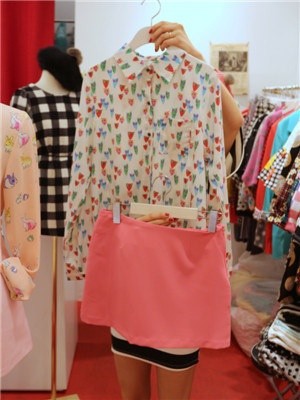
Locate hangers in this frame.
[85,1,300,234]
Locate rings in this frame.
[169,31,171,38]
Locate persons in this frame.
[1,102,38,378]
[111,21,245,400]
[10,46,85,237]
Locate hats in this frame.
[36,45,84,92]
